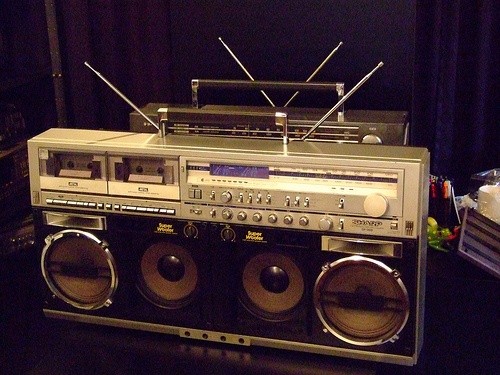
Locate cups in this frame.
[478,185,499,217]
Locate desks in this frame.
[2,226,500,375]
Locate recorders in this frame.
[26,61,430,367]
[128,34,413,147]
